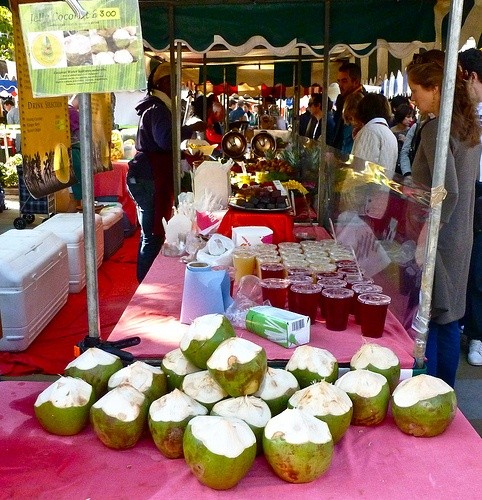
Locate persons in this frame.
[229,96,293,130]
[407,47,482,390]
[4,100,20,152]
[457,49,482,366]
[181,91,238,192]
[67,95,82,201]
[126,63,208,285]
[299,64,435,238]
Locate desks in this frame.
[0,226,482,500]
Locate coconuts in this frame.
[66,26,142,67]
[33,313,456,490]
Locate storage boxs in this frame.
[246,305,311,348]
[0,201,125,353]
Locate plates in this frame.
[229,200,292,212]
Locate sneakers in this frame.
[468,337,482,365]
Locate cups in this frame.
[231,239,391,339]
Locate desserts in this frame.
[237,190,286,208]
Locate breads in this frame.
[257,158,292,173]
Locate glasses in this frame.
[308,102,314,107]
[336,78,351,84]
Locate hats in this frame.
[153,63,177,81]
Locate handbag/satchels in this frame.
[192,159,235,211]
[181,171,193,193]
